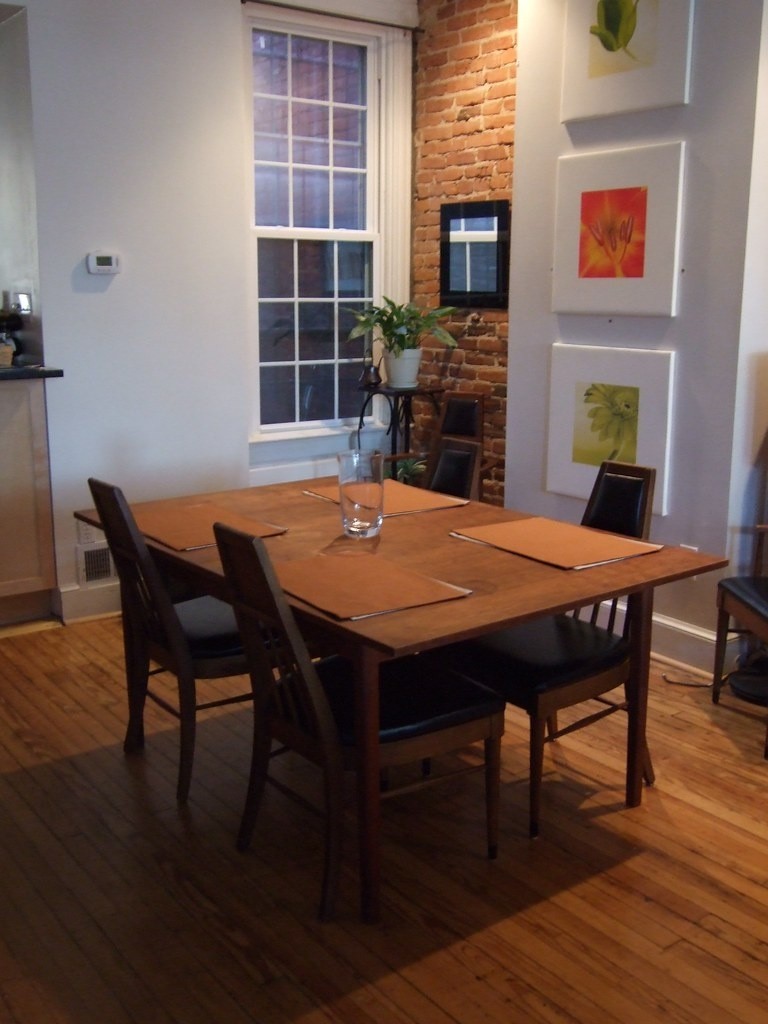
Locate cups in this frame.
[337,448,384,538]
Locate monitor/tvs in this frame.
[3,289,38,317]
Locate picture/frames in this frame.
[439,200,510,310]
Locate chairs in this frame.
[450,461,655,837]
[373,393,503,501]
[87,478,327,802]
[351,384,446,477]
[210,522,506,922]
[713,523,768,756]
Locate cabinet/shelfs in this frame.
[0,379,57,629]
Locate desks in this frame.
[73,475,728,925]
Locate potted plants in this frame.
[340,295,460,389]
